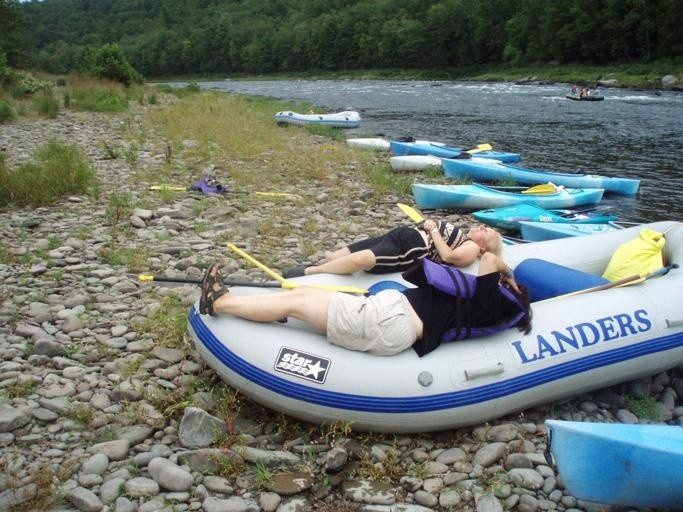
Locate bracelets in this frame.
[430,228,438,233]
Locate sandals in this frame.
[282,266,306,278]
[199,262,229,316]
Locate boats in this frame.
[441,157,641,196]
[346,135,444,154]
[389,139,521,162]
[472,202,618,231]
[411,181,605,210]
[564,90,604,102]
[517,219,648,245]
[389,152,501,175]
[274,109,361,130]
[543,418,682,510]
[185,218,682,436]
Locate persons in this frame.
[198,250,533,357]
[570,85,590,98]
[281,218,500,278]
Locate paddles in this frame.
[137,275,370,295]
[149,184,295,198]
[514,184,556,195]
[396,203,424,223]
